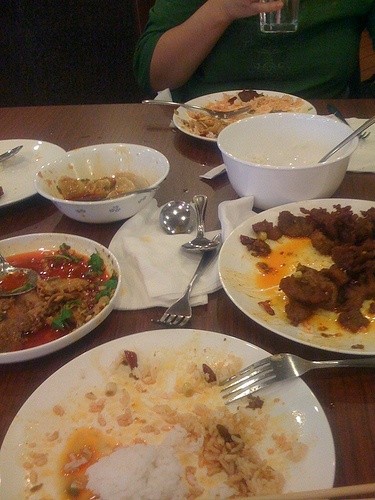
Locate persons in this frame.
[132,0,375,103]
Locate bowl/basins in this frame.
[217,112,359,210]
[34,143,171,224]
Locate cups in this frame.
[259,0,299,33]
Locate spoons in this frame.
[181,194,220,252]
[0,252,39,297]
[142,99,252,118]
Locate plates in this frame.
[172,89,318,142]
[0,232,122,364]
[0,328,337,500]
[0,139,66,207]
[160,201,196,235]
[217,198,375,357]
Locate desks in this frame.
[0,98,375,500]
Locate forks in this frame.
[217,353,375,404]
[327,104,371,139]
[151,231,222,327]
